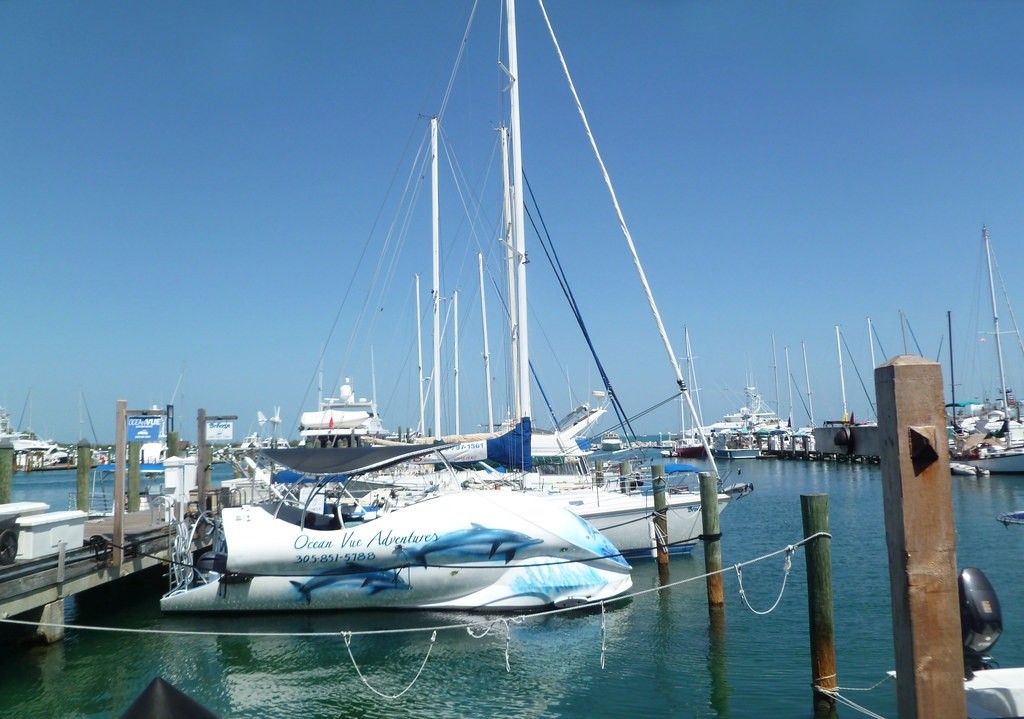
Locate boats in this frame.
[661,450,677,457]
[601,429,624,452]
[0,388,107,468]
[708,370,786,459]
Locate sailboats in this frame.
[160,0,754,614]
[759,219,1024,472]
[673,324,708,458]
